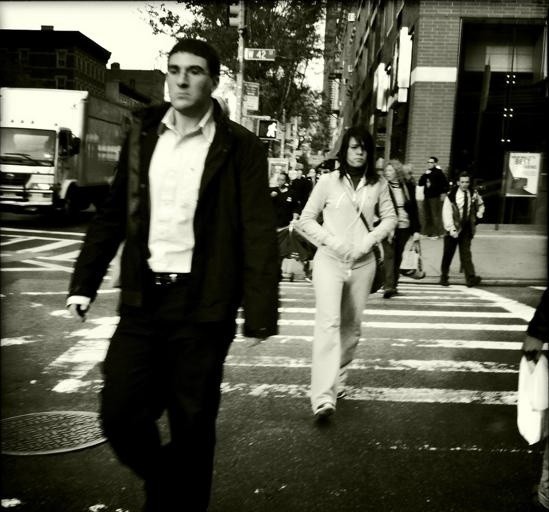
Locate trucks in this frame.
[0,86,149,217]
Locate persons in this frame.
[294,126,398,422]
[519,287,549,511]
[65,37,280,511]
[270,157,486,300]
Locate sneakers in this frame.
[143,441,173,510]
[313,390,346,417]
[384,288,397,298]
[440,276,481,287]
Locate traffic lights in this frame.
[255,118,277,141]
[228,1,243,29]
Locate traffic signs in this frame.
[243,48,275,62]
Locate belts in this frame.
[152,274,187,287]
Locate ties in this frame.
[463,191,468,223]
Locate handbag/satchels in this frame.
[517,353,549,445]
[400,242,425,279]
[370,261,385,294]
